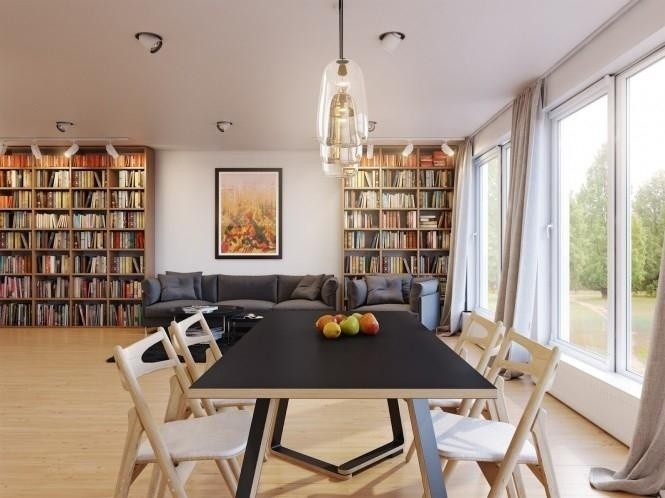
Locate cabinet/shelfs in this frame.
[2,145,155,330]
[342,146,457,302]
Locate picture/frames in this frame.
[215,166,283,258]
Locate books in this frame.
[0,150,148,330]
[345,139,460,308]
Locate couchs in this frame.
[349,276,442,335]
[137,270,339,335]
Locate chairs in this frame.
[404,328,564,497]
[170,313,269,462]
[114,327,252,497]
[402,309,502,441]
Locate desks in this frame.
[189,309,496,497]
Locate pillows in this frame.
[290,274,327,300]
[159,273,197,300]
[374,271,411,301]
[166,270,204,301]
[366,275,404,303]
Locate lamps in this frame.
[381,33,403,51]
[316,0,370,179]
[365,136,467,159]
[137,33,163,55]
[1,136,127,163]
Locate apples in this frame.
[316,312,380,338]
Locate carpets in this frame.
[107,330,231,363]
